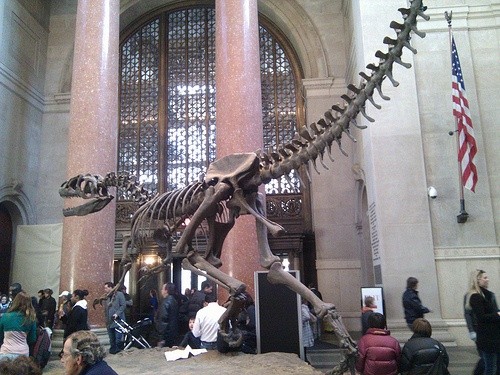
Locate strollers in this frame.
[109,315,156,354]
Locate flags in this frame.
[450,35,478,196]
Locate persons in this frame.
[102,281,134,354]
[148,280,255,351]
[402,277,430,332]
[61,330,119,375]
[301,296,315,365]
[463,269,500,375]
[307,283,323,339]
[400,318,451,375]
[0,283,91,375]
[354,312,401,375]
[361,297,378,335]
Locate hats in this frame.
[58,291,72,299]
